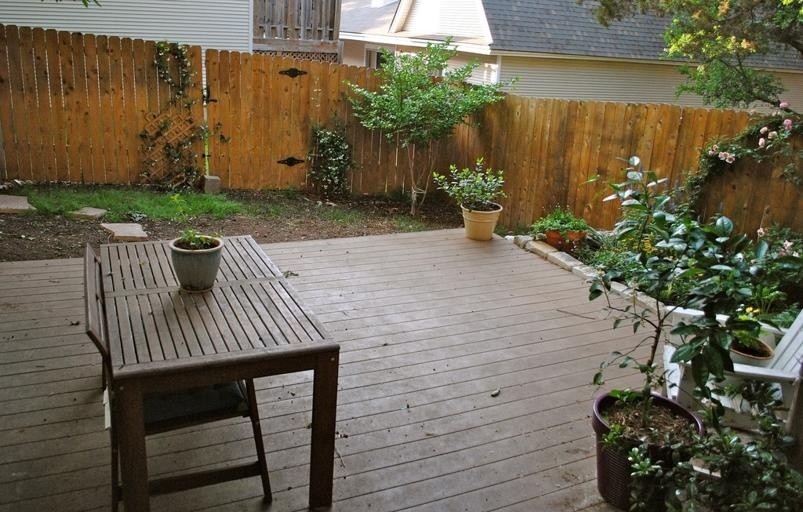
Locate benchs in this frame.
[81,238,275,512]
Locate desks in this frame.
[95,232,342,511]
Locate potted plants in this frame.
[433,152,510,239]
[165,191,225,294]
